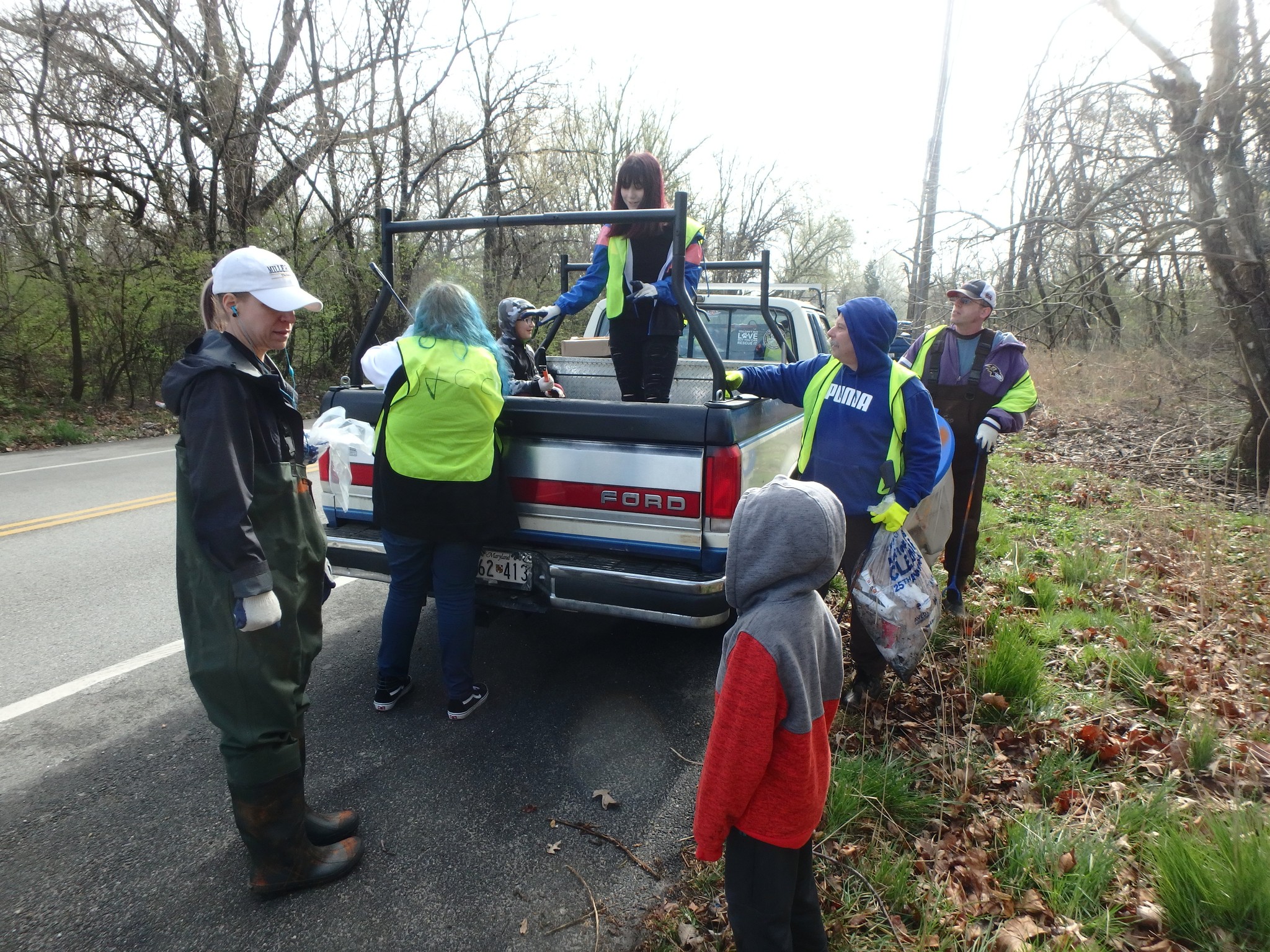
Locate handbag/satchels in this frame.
[900,458,953,567]
[850,491,942,685]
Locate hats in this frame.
[211,245,325,313]
[946,279,997,311]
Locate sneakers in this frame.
[373,671,412,711]
[447,682,489,720]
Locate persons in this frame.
[362,280,512,721]
[161,246,363,902]
[726,295,943,707]
[519,151,705,403]
[693,472,848,952]
[899,280,1038,616]
[497,296,565,398]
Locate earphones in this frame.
[231,305,238,315]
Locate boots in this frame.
[229,735,366,901]
[940,572,969,612]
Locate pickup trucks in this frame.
[311,192,834,641]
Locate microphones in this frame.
[288,365,295,377]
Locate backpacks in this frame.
[795,354,955,509]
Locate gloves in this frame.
[543,386,565,398]
[976,423,998,451]
[626,280,658,303]
[232,590,281,632]
[869,499,909,532]
[321,557,335,606]
[538,374,554,391]
[723,370,743,398]
[519,304,561,326]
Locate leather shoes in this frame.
[842,670,884,716]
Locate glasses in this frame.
[949,295,987,308]
[516,318,536,325]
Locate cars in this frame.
[888,321,914,361]
